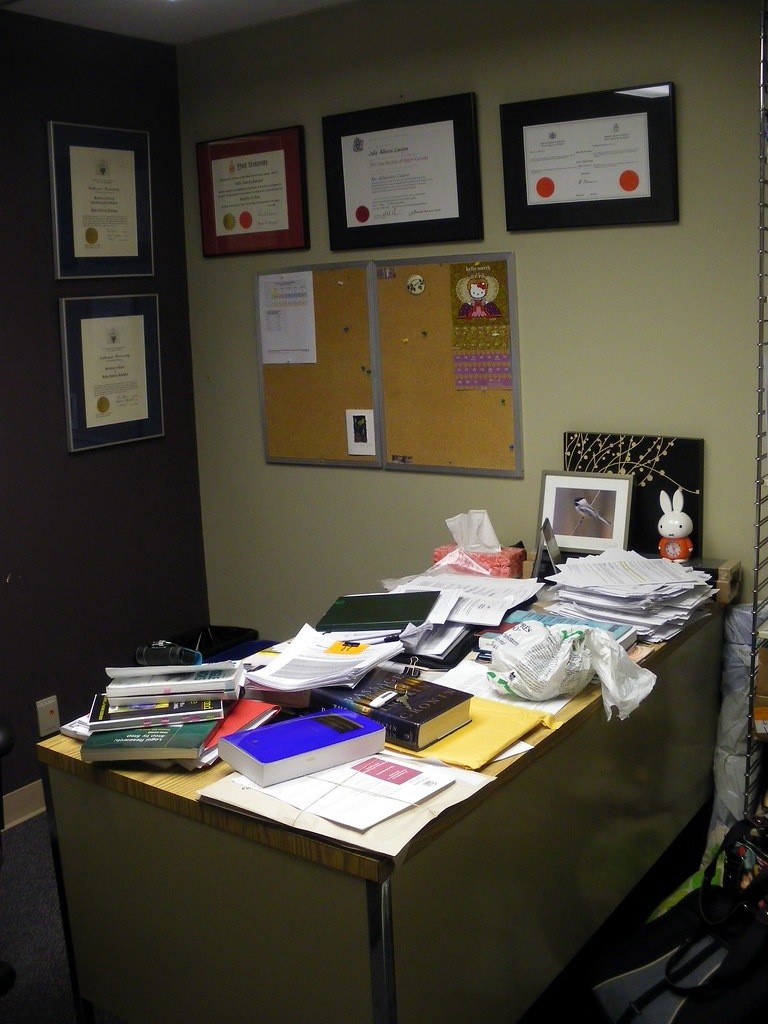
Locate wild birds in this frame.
[574,497,612,526]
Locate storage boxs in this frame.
[751,694,768,740]
[756,647,768,695]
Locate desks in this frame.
[34,597,725,1024]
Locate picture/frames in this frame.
[498,82,679,233]
[46,120,156,280]
[534,469,633,555]
[60,292,165,453]
[321,92,485,251]
[196,124,310,256]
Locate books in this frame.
[316,590,442,632]
[310,663,473,752]
[58,661,282,769]
[473,610,638,685]
[217,709,384,788]
